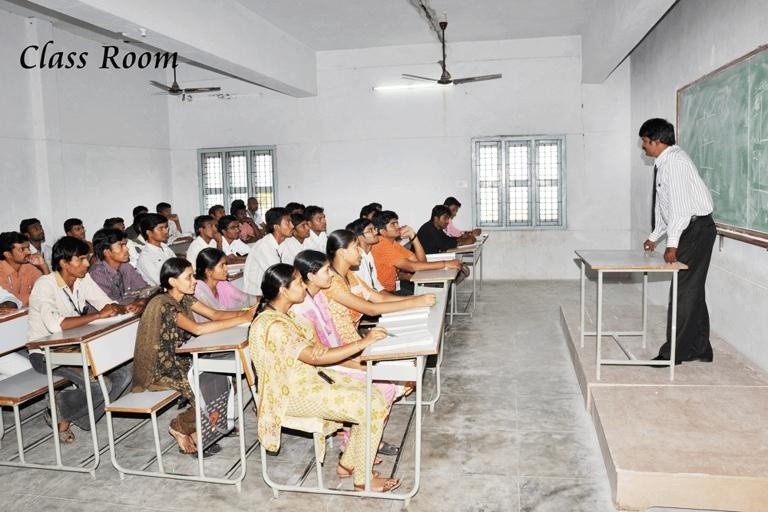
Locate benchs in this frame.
[1,314,70,468]
[242,345,324,494]
[86,321,181,480]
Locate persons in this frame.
[86,227,152,316]
[185,215,222,274]
[247,263,401,494]
[243,207,294,295]
[0,286,22,317]
[130,257,259,454]
[347,217,394,322]
[212,215,250,256]
[411,205,475,298]
[124,205,149,241]
[137,214,177,287]
[0,231,50,307]
[369,210,460,295]
[231,199,244,206]
[103,217,125,231]
[288,250,400,465]
[283,211,314,268]
[27,235,126,444]
[156,202,193,246]
[370,203,382,210]
[441,196,483,237]
[229,202,264,244]
[304,205,329,254]
[320,229,434,402]
[19,218,53,269]
[246,196,266,235]
[63,218,93,253]
[285,203,304,214]
[360,205,375,220]
[638,117,717,368]
[191,247,263,324]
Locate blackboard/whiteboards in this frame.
[675,43,768,249]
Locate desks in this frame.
[572,249,688,378]
[447,233,490,314]
[411,254,464,329]
[174,305,255,483]
[27,311,140,471]
[362,286,449,502]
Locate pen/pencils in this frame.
[367,327,396,338]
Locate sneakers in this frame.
[200,442,222,452]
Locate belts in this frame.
[691,215,716,222]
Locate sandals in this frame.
[376,440,402,455]
[336,464,380,478]
[41,408,76,444]
[338,452,384,465]
[45,390,65,427]
[353,478,401,492]
[180,447,212,458]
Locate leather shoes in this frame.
[647,354,684,368]
[687,352,716,364]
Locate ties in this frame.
[651,165,659,235]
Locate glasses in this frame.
[227,224,241,230]
[358,227,378,235]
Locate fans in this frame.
[401,18,503,86]
[149,54,223,97]
